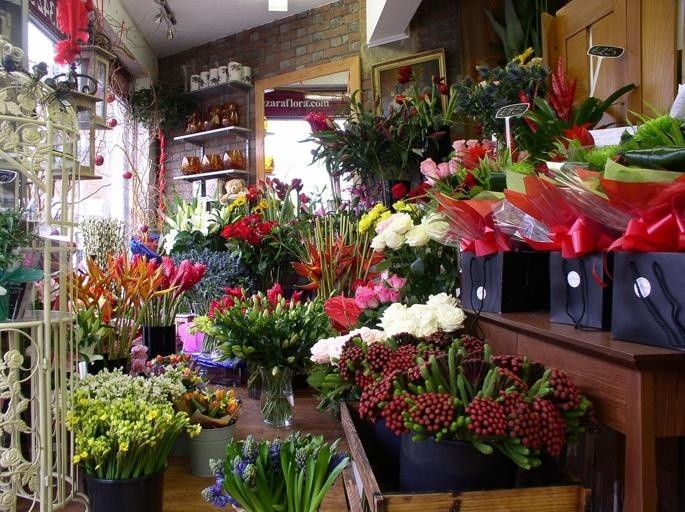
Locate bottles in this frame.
[183,101,245,173]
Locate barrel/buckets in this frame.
[187,422,236,478]
[80,466,169,512]
[398,429,525,494]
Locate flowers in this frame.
[302,47,683,169]
[65,168,683,512]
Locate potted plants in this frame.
[0,204,38,321]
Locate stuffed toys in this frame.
[219,178,249,210]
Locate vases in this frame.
[181,149,245,175]
[181,62,252,93]
[184,100,239,134]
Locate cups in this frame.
[181,63,254,90]
